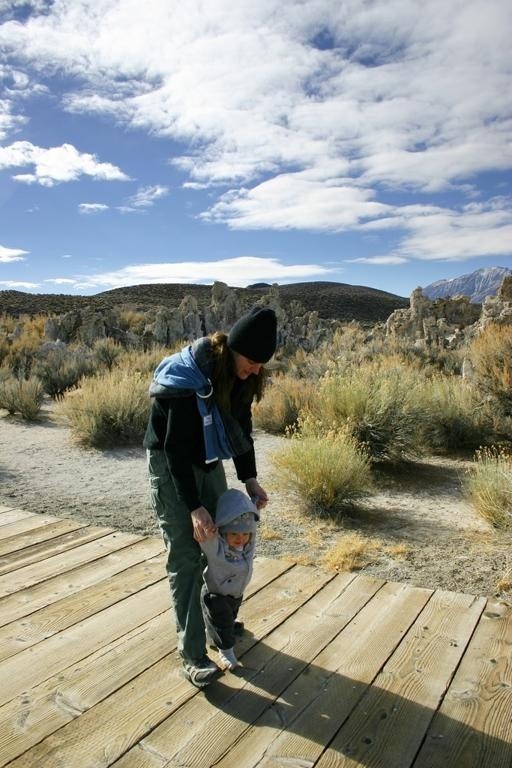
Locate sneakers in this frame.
[180,618,245,687]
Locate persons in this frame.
[145,306,279,690]
[191,487,264,673]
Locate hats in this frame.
[219,513,257,536]
[225,302,277,364]
